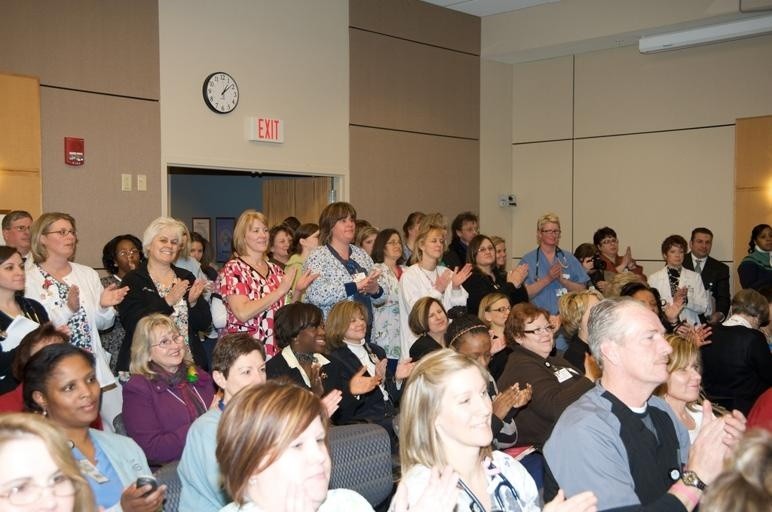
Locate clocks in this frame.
[203,71,239,114]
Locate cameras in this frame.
[591,257,609,271]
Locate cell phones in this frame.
[137,476,157,492]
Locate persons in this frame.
[0,201,771,511]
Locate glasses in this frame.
[490,305,513,313]
[514,322,555,337]
[541,229,562,235]
[147,333,185,352]
[383,240,403,246]
[0,477,83,504]
[42,228,76,237]
[7,224,30,232]
[115,248,140,260]
[476,245,495,253]
[600,238,618,245]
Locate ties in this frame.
[694,259,703,274]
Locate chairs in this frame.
[151,462,183,512]
[322,424,393,508]
